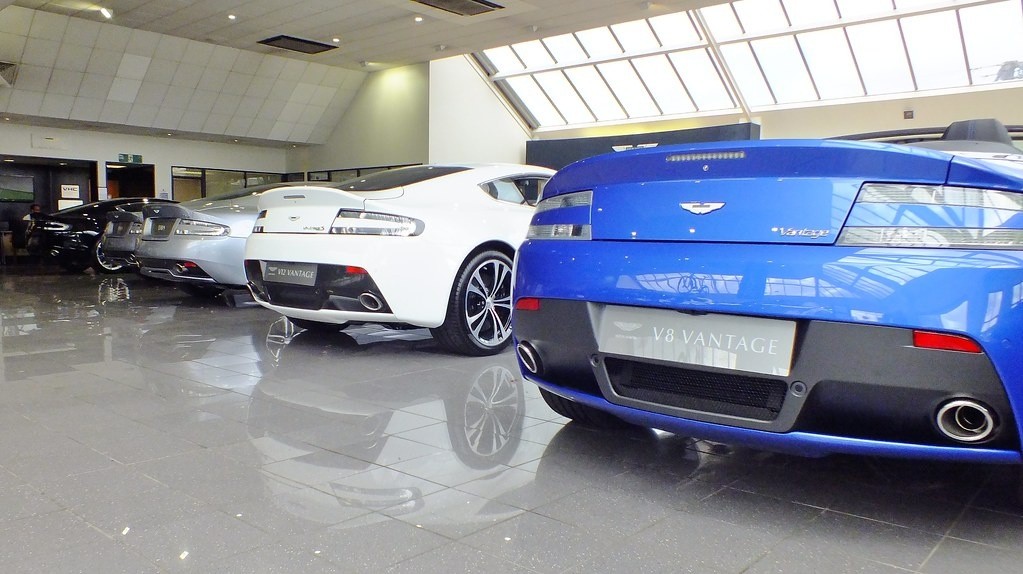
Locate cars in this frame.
[246,325,663,532]
[243,161,559,357]
[512,118,1023,464]
[26,197,181,274]
[102,182,333,287]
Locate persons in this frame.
[7,203,40,275]
